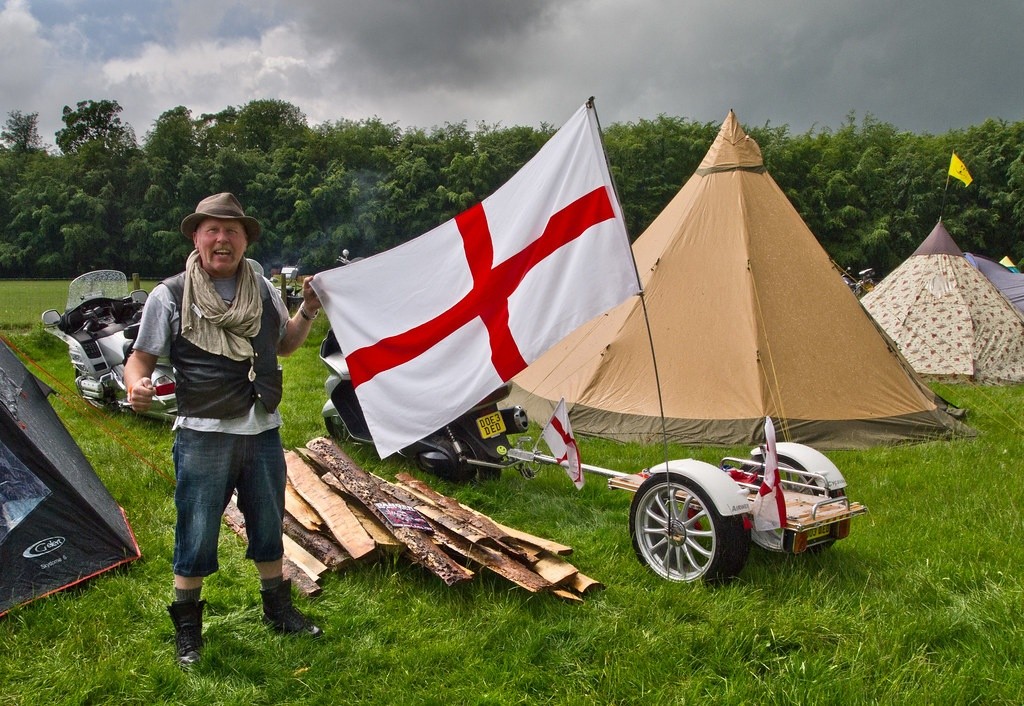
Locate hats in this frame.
[181,193,261,247]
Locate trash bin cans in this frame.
[271,269,281,275]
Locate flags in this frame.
[949,154,973,187]
[308,106,643,460]
[541,397,586,490]
[753,416,787,530]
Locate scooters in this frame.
[319,249,530,486]
[269,276,295,308]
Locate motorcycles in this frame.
[42,271,180,416]
[840,266,876,298]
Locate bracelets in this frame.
[300,302,319,321]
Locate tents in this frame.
[496,111,978,452]
[963,253,1024,314]
[860,223,1024,385]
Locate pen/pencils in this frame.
[153,394,166,406]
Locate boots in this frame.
[260,579,324,639]
[166,598,206,673]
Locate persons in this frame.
[124,193,331,671]
